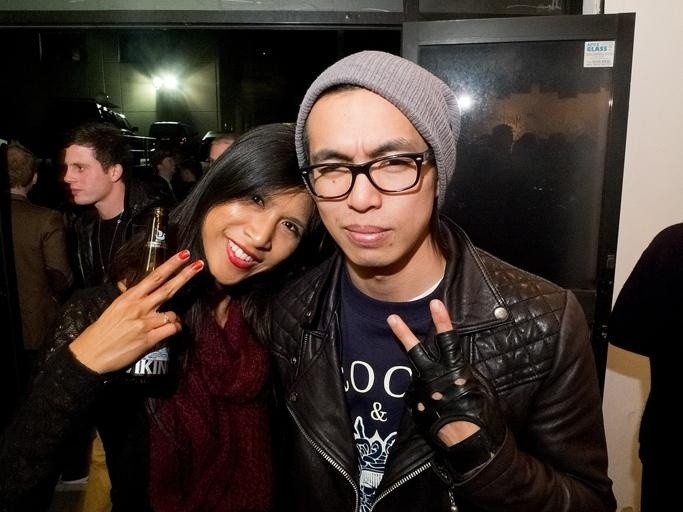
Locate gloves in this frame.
[401,327,511,474]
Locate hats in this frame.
[293,46,463,214]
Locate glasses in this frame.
[296,145,436,199]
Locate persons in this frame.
[0,125,241,512]
[258,50,615,511]
[23,122,326,511]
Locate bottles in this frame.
[109,203,178,400]
